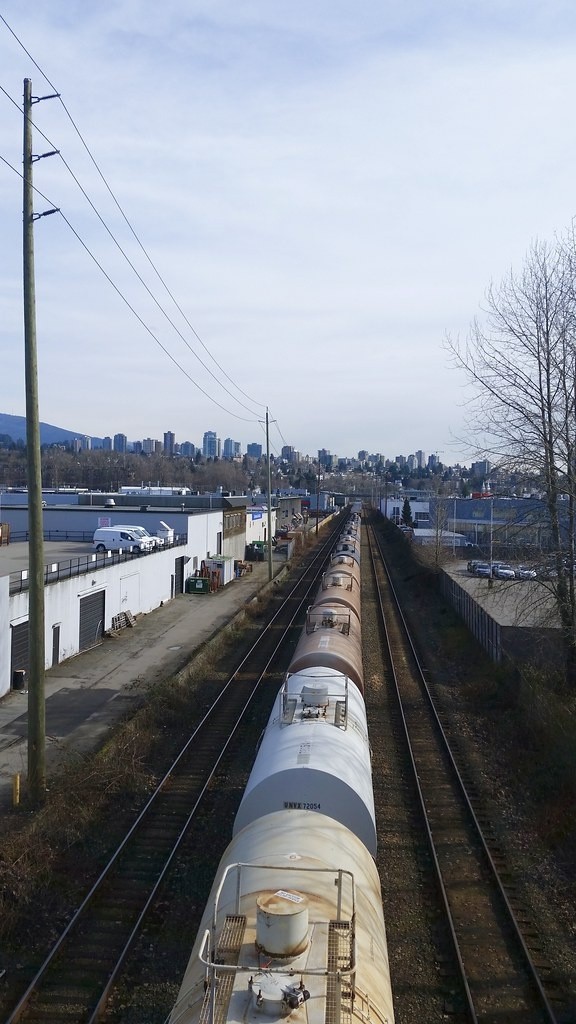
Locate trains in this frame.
[163,497,395,1024]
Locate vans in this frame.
[93,524,164,554]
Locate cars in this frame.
[467,559,558,582]
